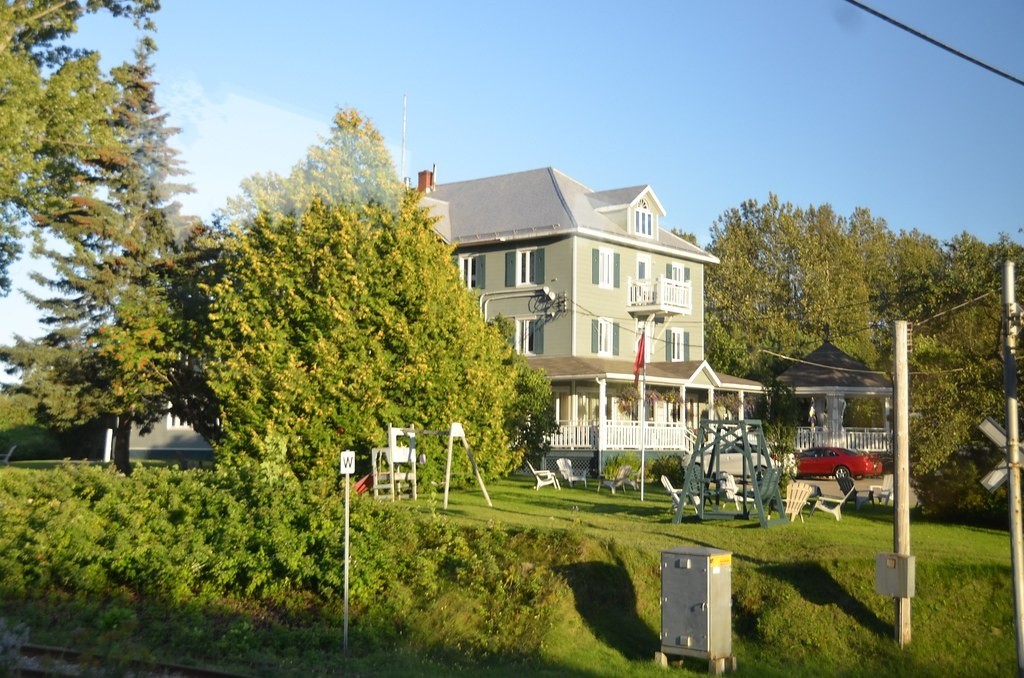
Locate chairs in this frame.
[557,458,587,488]
[526,460,561,492]
[837,478,875,512]
[869,474,894,509]
[624,465,642,491]
[603,465,633,494]
[372,423,418,504]
[721,475,757,513]
[659,475,700,515]
[810,485,855,521]
[767,482,812,523]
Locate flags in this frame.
[632,331,644,385]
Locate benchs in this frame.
[685,466,726,499]
[734,467,782,505]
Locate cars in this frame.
[678,440,784,484]
[792,444,882,479]
